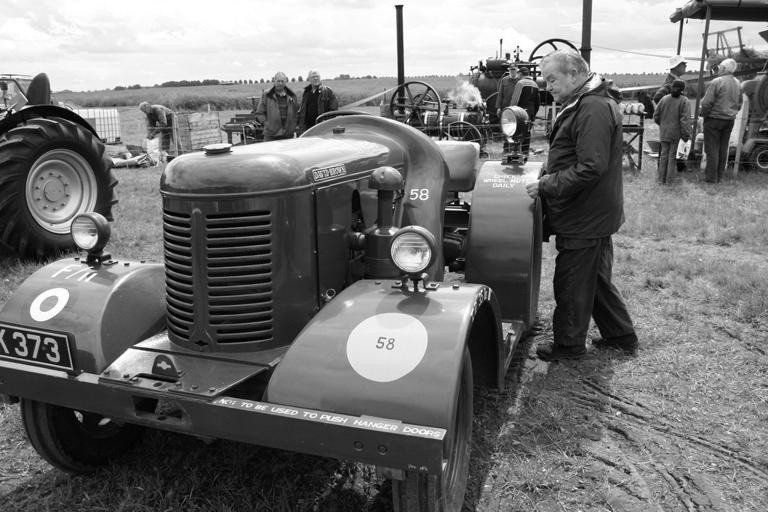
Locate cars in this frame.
[423,37,581,149]
[671,1,767,172]
[0,4,546,512]
[0,72,118,260]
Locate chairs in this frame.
[434,140,477,214]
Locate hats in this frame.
[669,55,688,70]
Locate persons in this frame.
[526,46,640,363]
[653,78,695,185]
[496,63,519,160]
[654,55,688,167]
[255,71,296,141]
[296,70,339,137]
[509,66,541,162]
[139,101,177,151]
[698,57,743,184]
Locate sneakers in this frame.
[592,334,637,350]
[537,341,587,360]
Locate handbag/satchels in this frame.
[676,138,692,160]
